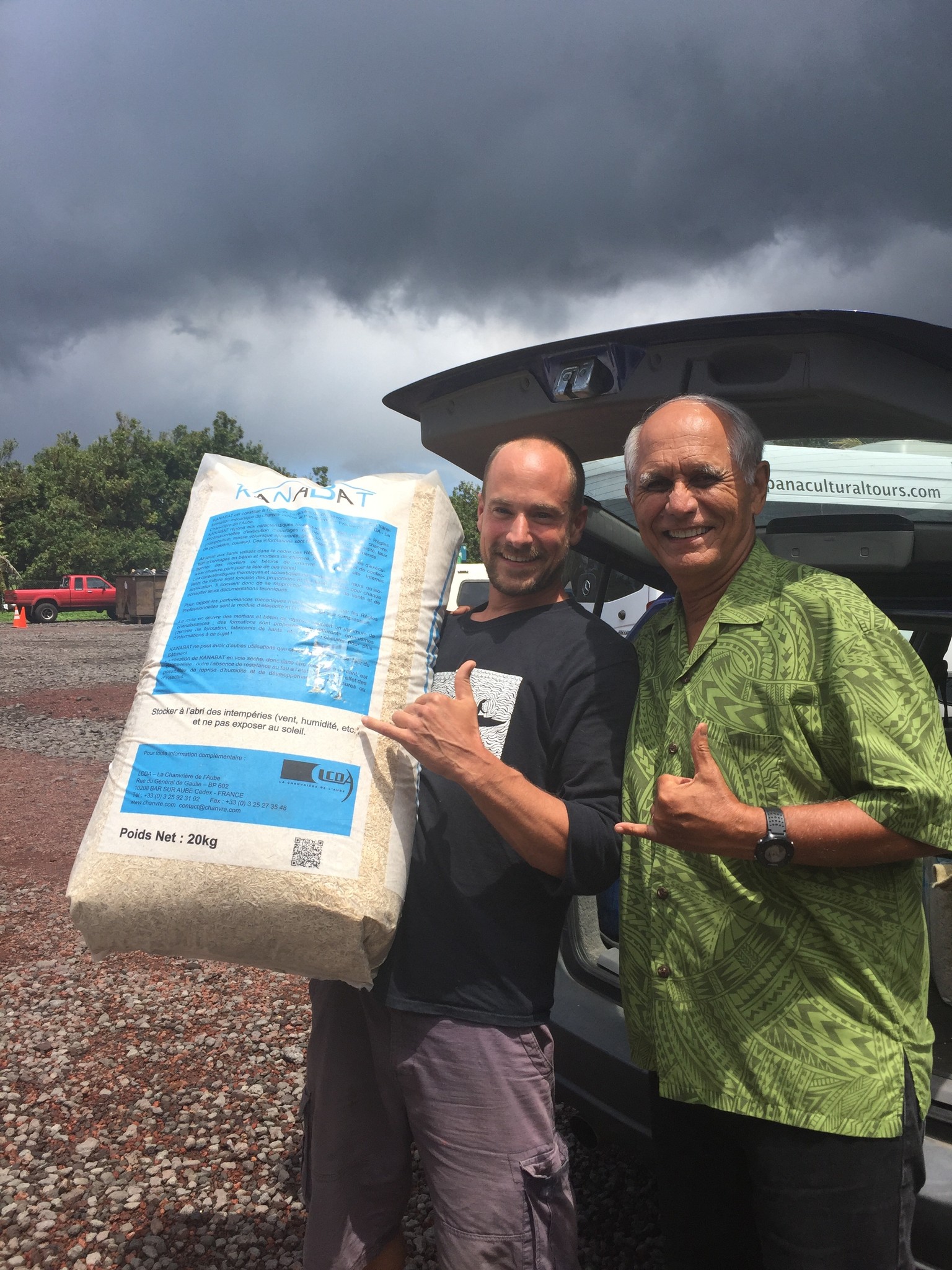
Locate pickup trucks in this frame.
[4,575,120,624]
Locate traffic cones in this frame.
[15,607,29,628]
[10,606,20,628]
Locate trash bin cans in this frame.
[113,574,168,624]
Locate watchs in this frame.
[750,806,797,873]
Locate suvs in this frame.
[380,309,951,1270]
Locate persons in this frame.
[451,396,952,1270]
[300,432,646,1270]
